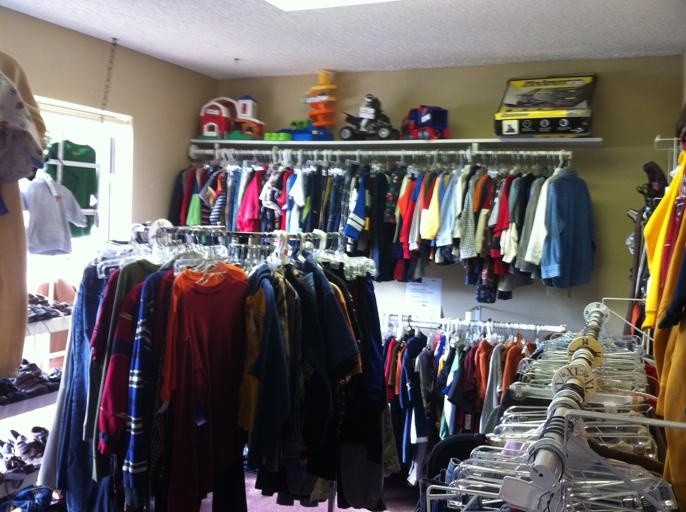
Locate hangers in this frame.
[427,328,675,512]
[83,216,376,291]
[187,147,569,181]
[387,311,542,353]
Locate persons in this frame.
[358,94,376,131]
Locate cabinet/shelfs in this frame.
[0,316,71,500]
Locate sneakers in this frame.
[27,294,72,322]
[0,427,49,480]
[0,359,61,404]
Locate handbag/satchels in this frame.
[0,486,64,511]
